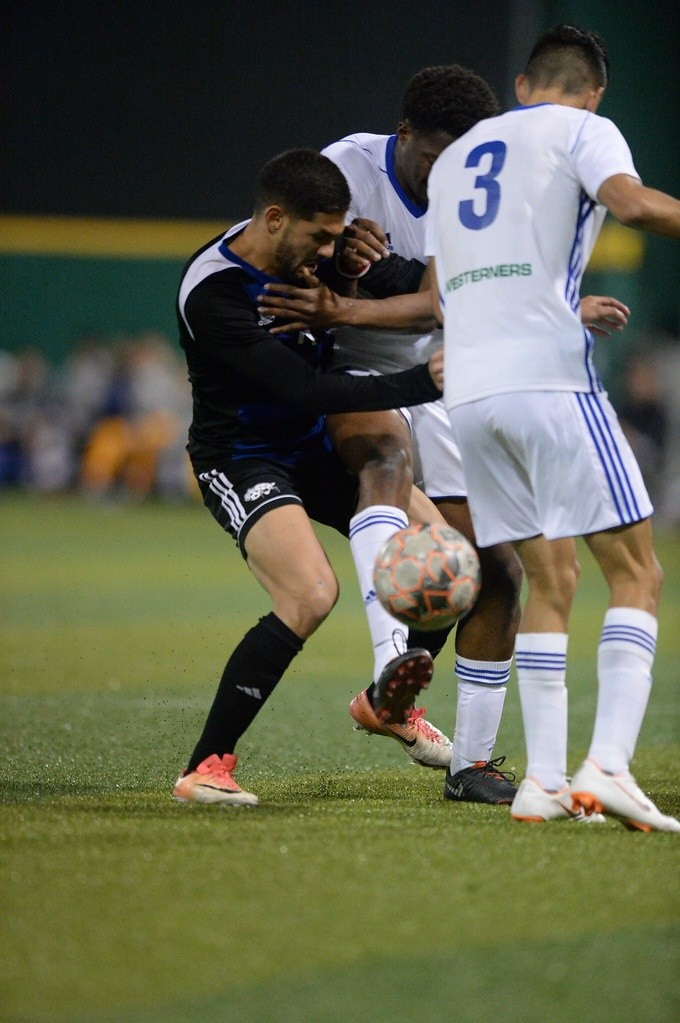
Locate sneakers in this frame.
[509,778,607,825]
[373,648,434,724]
[443,755,519,806]
[169,753,261,808]
[570,759,680,833]
[348,688,454,770]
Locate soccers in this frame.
[371,522,482,632]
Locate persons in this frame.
[175,146,443,808]
[1,335,198,501]
[614,354,667,480]
[427,24,680,834]
[257,65,521,806]
